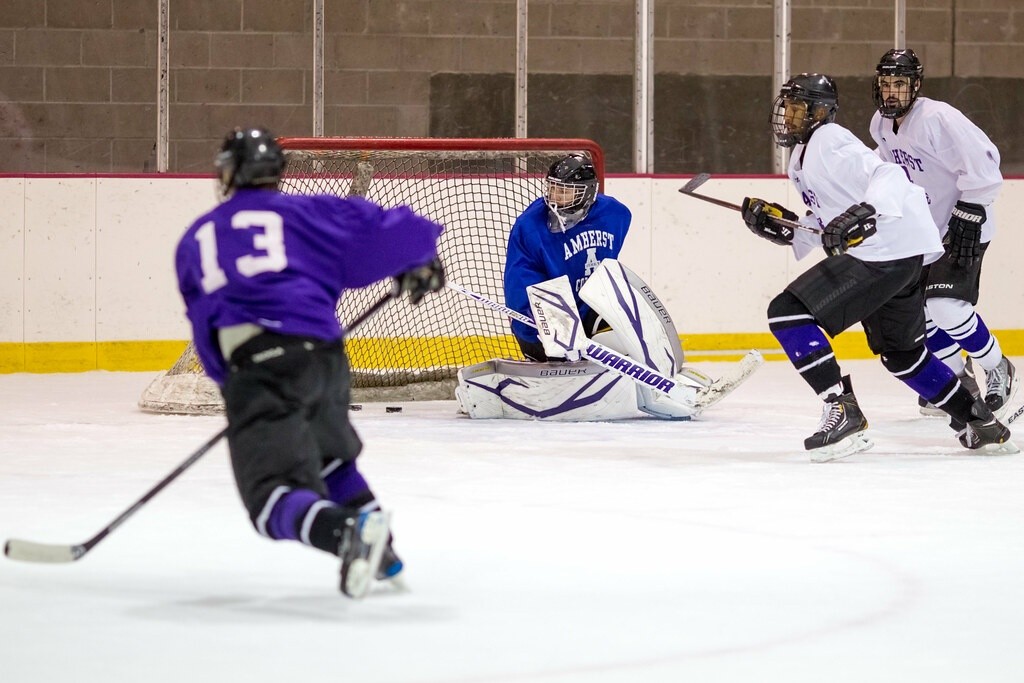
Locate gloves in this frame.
[822,202,876,254]
[390,253,445,304]
[742,196,799,245]
[937,199,986,269]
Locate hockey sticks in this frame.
[3,284,400,567]
[677,172,823,237]
[441,281,763,418]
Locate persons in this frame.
[504,152,714,421]
[742,48,1019,449]
[174,127,445,598]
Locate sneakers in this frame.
[981,354,1021,421]
[802,373,875,464]
[947,391,1021,457]
[296,490,403,600]
[918,354,981,418]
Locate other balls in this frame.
[385,406,403,414]
[346,403,363,411]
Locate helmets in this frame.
[870,48,925,120]
[214,123,285,203]
[540,151,600,215]
[767,71,840,150]
